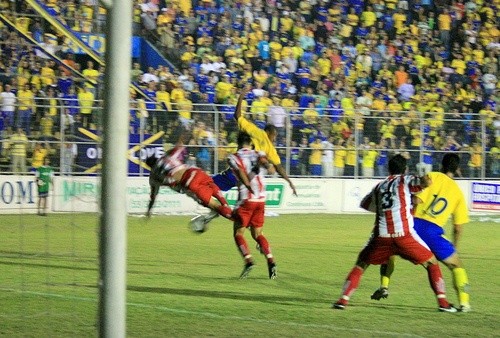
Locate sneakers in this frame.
[334,297,347,308]
[268,263,277,280]
[256,244,262,252]
[371,288,389,300]
[438,304,462,313]
[240,261,255,280]
[461,305,471,312]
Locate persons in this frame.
[0,0,500,177]
[372,152,471,313]
[34,156,55,216]
[144,128,232,220]
[334,154,461,314]
[190,85,298,234]
[227,132,278,281]
